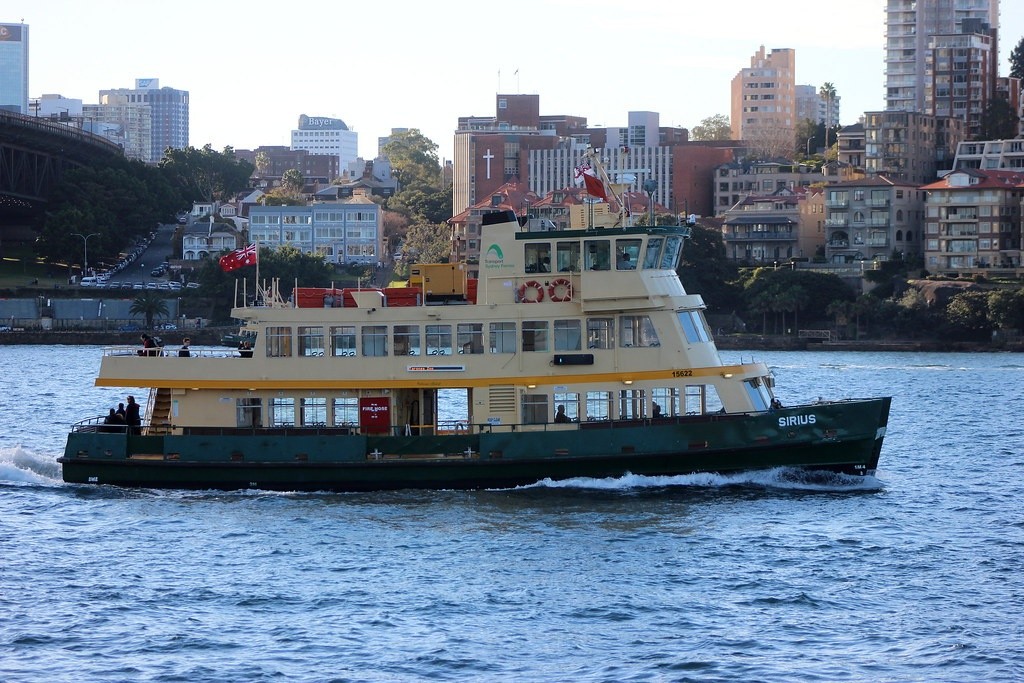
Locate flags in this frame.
[220,241,257,272]
[573,163,609,204]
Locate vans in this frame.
[80,277,98,287]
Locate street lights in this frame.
[70,232,101,276]
[177,296,181,330]
[141,263,146,287]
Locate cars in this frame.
[110,281,120,289]
[117,323,139,331]
[186,282,199,289]
[174,226,179,233]
[150,261,169,277]
[0,324,12,333]
[96,232,156,281]
[152,322,177,330]
[133,281,146,289]
[169,281,180,289]
[97,281,108,288]
[159,284,170,290]
[121,281,133,288]
[145,282,158,288]
[179,217,186,224]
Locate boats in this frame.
[51,140,893,494]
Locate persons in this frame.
[541,253,635,273]
[555,405,571,422]
[652,402,661,418]
[103,333,253,436]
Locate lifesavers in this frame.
[519,281,545,302]
[548,278,574,302]
